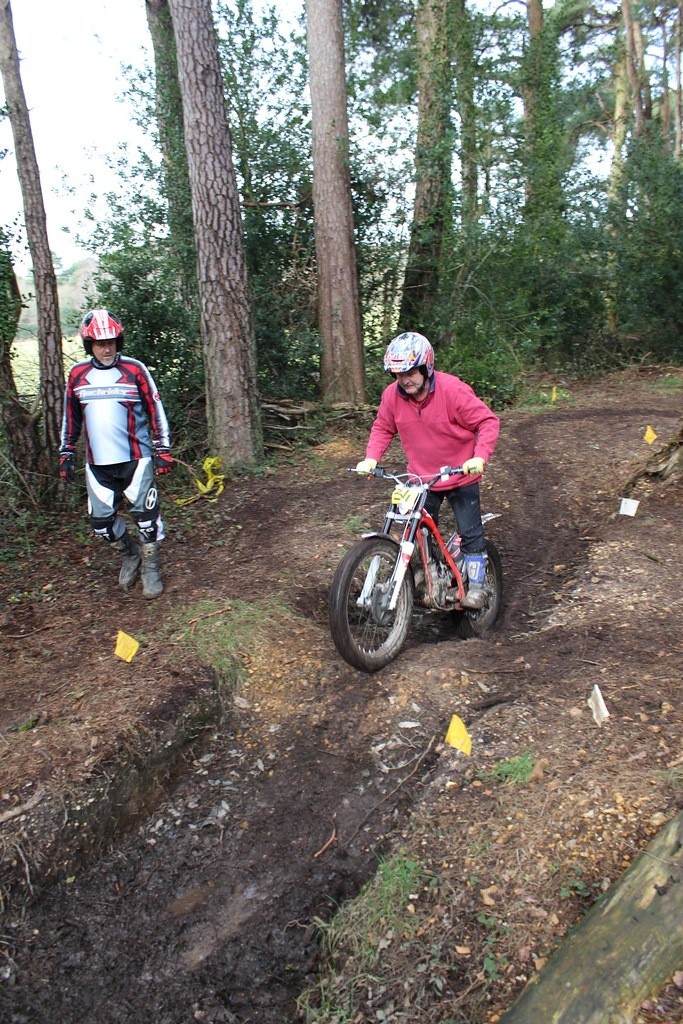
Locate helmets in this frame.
[82,309,125,357]
[383,332,434,379]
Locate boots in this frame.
[459,551,490,609]
[108,527,142,590]
[137,542,164,599]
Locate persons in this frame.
[58,309,172,600]
[358,331,502,609]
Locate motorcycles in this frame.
[327,466,504,672]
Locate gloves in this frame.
[153,448,177,476]
[57,450,75,480]
[462,457,485,477]
[356,458,377,480]
